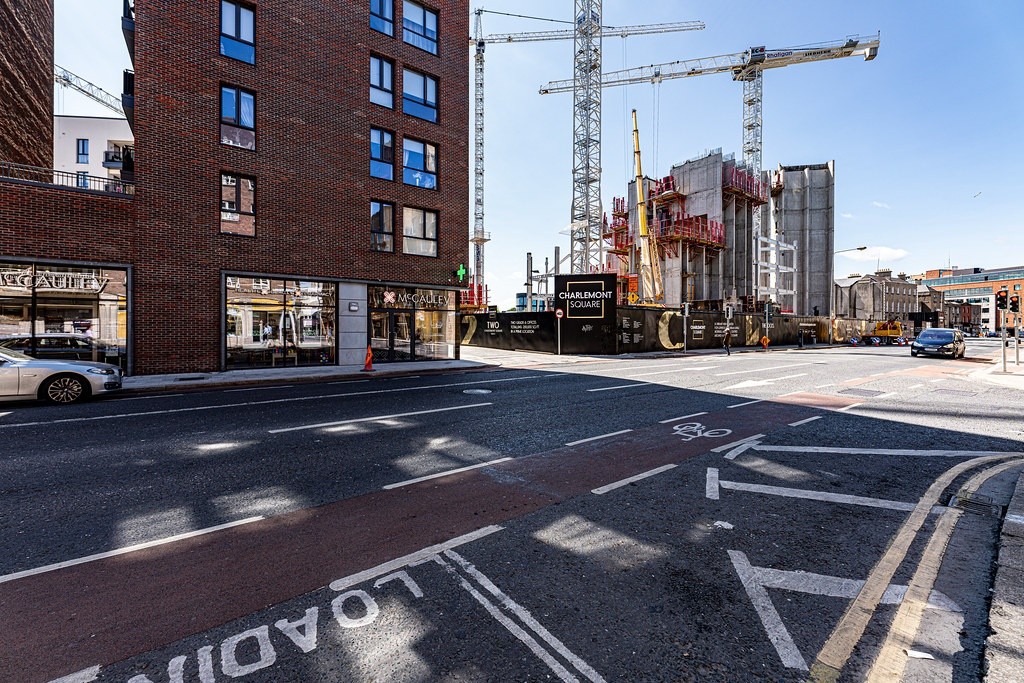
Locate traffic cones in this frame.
[359,345,376,372]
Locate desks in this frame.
[272,353,298,366]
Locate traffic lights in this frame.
[1009,296,1020,313]
[996,289,1009,311]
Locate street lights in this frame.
[828,245,867,346]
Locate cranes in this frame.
[537,30,882,206]
[469,0,705,319]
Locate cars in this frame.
[910,327,966,360]
[0,344,124,405]
[0,333,127,370]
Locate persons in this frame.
[85,325,93,341]
[261,323,272,344]
[722,328,731,356]
[327,325,332,343]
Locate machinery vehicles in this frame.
[860,315,904,345]
